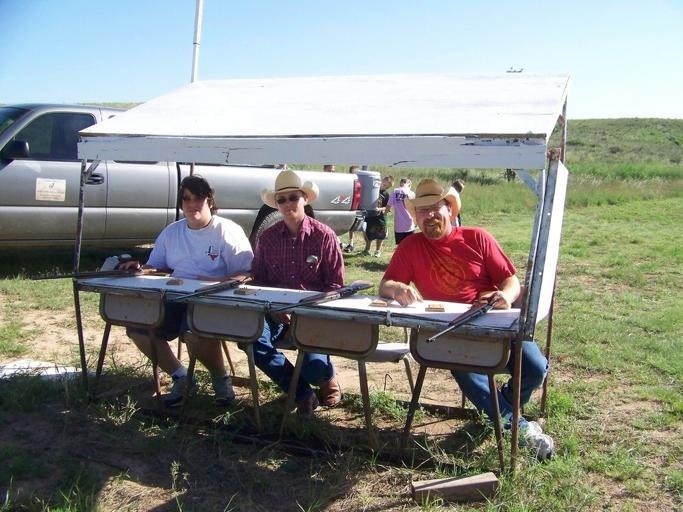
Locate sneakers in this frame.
[163,374,197,407]
[374,249,381,258]
[525,421,553,461]
[344,244,354,253]
[213,375,235,405]
[357,250,371,256]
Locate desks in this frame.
[77,271,523,479]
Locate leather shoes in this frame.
[295,392,320,415]
[319,376,342,406]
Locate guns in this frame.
[266,280,374,315]
[167,273,254,304]
[425,297,493,343]
[31,264,158,281]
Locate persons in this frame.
[384,178,416,244]
[344,166,367,252]
[450,179,464,227]
[118,175,254,409]
[229,169,345,421]
[356,175,394,258]
[378,178,555,464]
[323,165,334,172]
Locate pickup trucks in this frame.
[0,104,364,260]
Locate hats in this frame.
[260,170,319,209]
[404,178,462,225]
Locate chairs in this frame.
[361,325,415,401]
[178,337,235,377]
[398,286,525,470]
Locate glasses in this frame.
[276,193,298,204]
[183,195,205,202]
